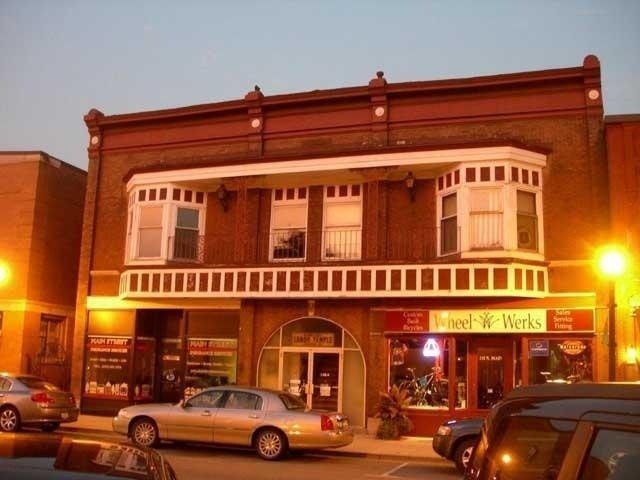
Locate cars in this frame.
[113,386,354,460]
[433,382,640,480]
[0,432,176,480]
[0,372,79,432]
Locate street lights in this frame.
[600,252,622,382]
